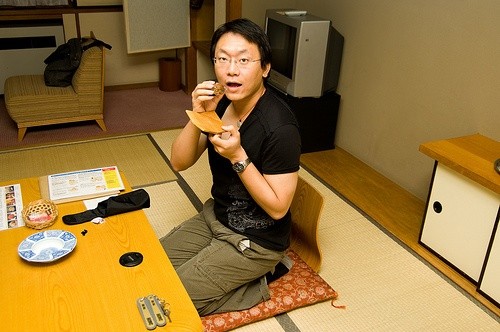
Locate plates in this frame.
[17,230,76,263]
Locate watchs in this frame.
[232,157,252,173]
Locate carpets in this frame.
[0,127,500,332]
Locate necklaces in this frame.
[236,91,263,127]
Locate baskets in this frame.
[21,197,58,230]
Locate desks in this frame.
[0,171,207,332]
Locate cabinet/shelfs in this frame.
[267,86,341,154]
[123,0,243,96]
[418,134,500,308]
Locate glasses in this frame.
[212,55,261,67]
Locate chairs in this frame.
[5,31,107,141]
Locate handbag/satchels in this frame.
[42,29,112,87]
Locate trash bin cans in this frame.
[157,56,182,92]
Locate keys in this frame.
[157,298,172,323]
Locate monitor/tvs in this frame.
[263,9,344,99]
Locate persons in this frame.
[159,18,302,318]
